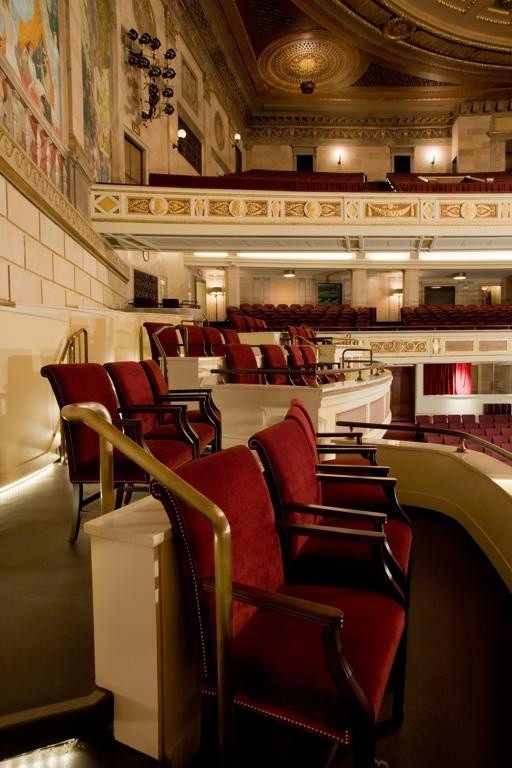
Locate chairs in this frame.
[224,329,260,347]
[179,325,207,356]
[148,445,408,768]
[143,322,180,357]
[284,345,322,385]
[226,303,512,332]
[417,414,511,460]
[248,419,414,579]
[300,346,345,381]
[142,360,221,451]
[221,344,260,383]
[40,363,193,542]
[285,407,396,502]
[204,327,226,356]
[291,398,376,464]
[287,326,333,345]
[104,361,217,458]
[260,345,296,385]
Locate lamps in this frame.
[125,26,178,134]
